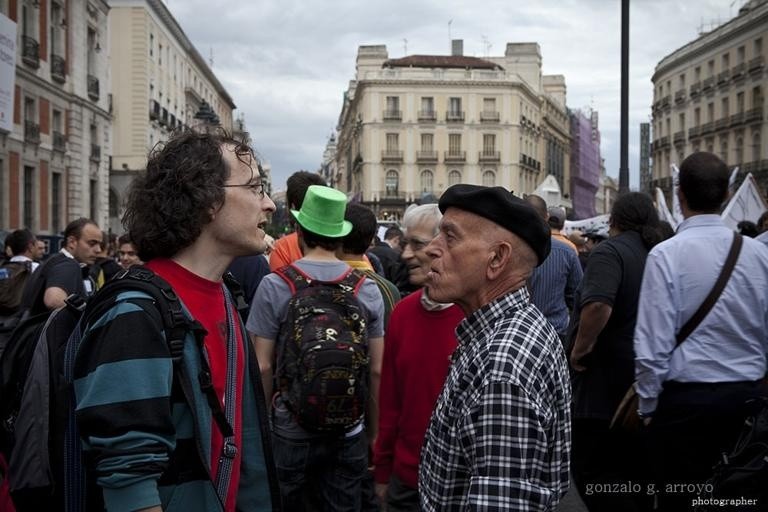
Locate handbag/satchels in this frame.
[606,375,659,446]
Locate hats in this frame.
[290,184,354,241]
[582,230,611,241]
[544,209,567,231]
[439,179,556,271]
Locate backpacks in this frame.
[16,263,118,512]
[270,261,371,433]
[0,258,33,320]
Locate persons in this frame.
[66,120,286,510]
[411,181,574,511]
[628,151,768,512]
[1,171,767,510]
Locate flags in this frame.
[668,164,685,224]
[721,167,740,210]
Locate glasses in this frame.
[397,236,441,251]
